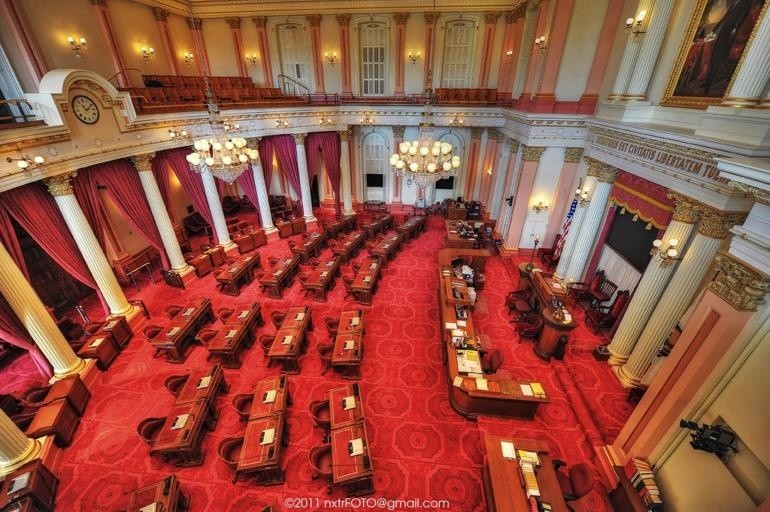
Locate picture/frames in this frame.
[660,1,770,110]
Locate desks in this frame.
[0,454,61,512]
[443,199,479,247]
[477,431,569,511]
[172,296,216,332]
[76,316,133,373]
[327,384,376,499]
[332,308,367,380]
[121,473,188,511]
[24,373,91,448]
[396,216,427,244]
[607,463,648,511]
[437,247,553,422]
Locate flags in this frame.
[553,199,577,260]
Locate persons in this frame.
[456,263,474,287]
[684,0,752,81]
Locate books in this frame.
[516,450,553,511]
[623,457,664,511]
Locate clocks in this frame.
[71,94,101,126]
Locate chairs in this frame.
[537,232,565,272]
[69,339,86,353]
[184,202,410,302]
[119,74,283,110]
[456,87,498,105]
[505,288,546,344]
[6,386,50,432]
[565,264,630,336]
[549,457,595,502]
[478,348,504,373]
[135,302,343,496]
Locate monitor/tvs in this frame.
[367,174,383,187]
[436,175,454,189]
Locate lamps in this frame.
[68,34,89,60]
[325,52,337,64]
[6,148,45,171]
[387,2,463,189]
[141,46,154,63]
[623,9,648,39]
[535,35,547,55]
[652,236,684,268]
[574,186,591,207]
[184,0,261,188]
